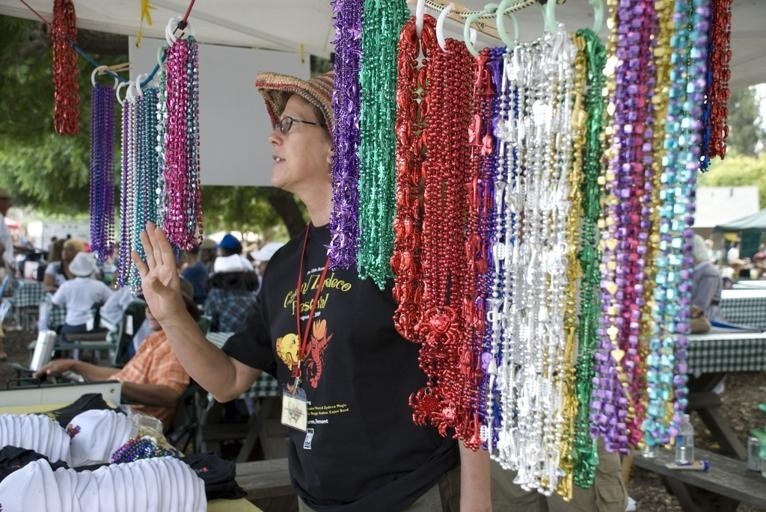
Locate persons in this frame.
[1,71,765,511]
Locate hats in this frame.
[249,58,343,144]
[199,237,217,251]
[0,410,212,512]
[212,253,254,273]
[66,249,99,278]
[134,271,208,318]
[0,186,13,201]
[247,240,285,264]
[214,232,241,252]
[60,238,84,261]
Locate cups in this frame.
[748,436,762,472]
[29,330,57,370]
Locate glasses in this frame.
[271,115,322,135]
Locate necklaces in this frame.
[330,0,731,505]
[53,0,201,292]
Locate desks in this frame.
[633,277,766,457]
[0,398,268,512]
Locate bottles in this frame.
[642,415,661,457]
[676,412,693,464]
[665,459,708,473]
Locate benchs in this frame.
[604,424,765,512]
